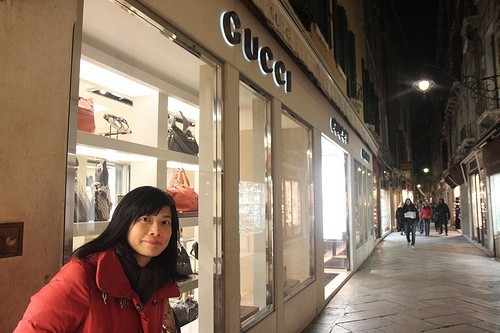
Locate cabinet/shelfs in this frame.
[73,129,198,295]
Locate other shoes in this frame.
[439,232,443,234]
[407,242,411,247]
[446,233,448,236]
[410,245,416,249]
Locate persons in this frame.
[14,186,179,333]
[396,197,461,249]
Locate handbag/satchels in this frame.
[430,216,434,222]
